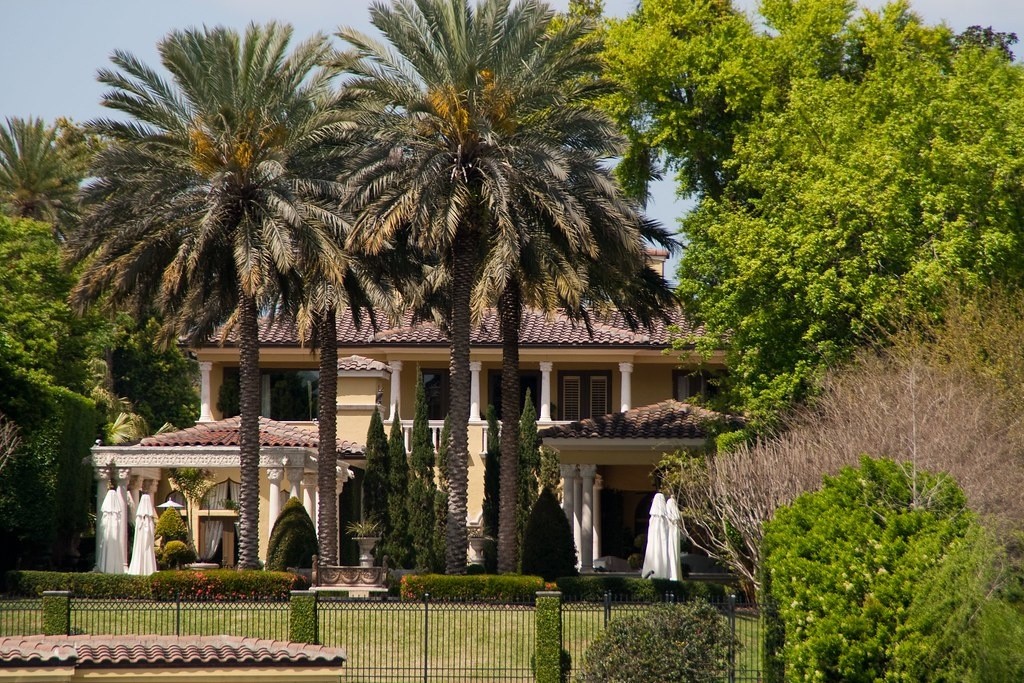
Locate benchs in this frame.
[310,555,390,601]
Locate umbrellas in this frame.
[666,498,683,582]
[128,494,158,575]
[641,493,671,580]
[98,486,136,575]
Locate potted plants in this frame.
[469,528,493,564]
[345,519,383,567]
